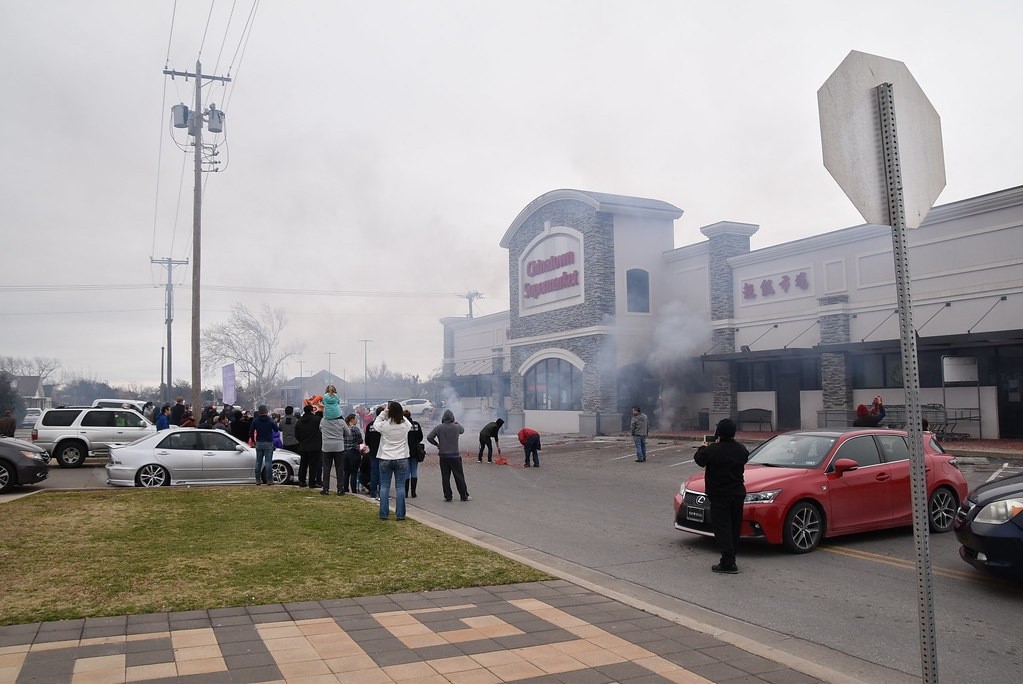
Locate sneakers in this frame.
[712,561,739,573]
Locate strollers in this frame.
[356,452,378,492]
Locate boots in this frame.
[410,477,418,497]
[404,478,410,498]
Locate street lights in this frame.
[161,347,164,382]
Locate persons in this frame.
[365,407,392,500]
[363,408,373,434]
[403,410,423,499]
[630,405,649,462]
[0,409,16,437]
[359,453,371,495]
[373,402,413,521]
[344,414,363,493]
[323,385,344,420]
[117,401,153,426]
[180,410,196,427]
[319,418,351,496]
[155,396,185,431]
[852,395,885,427]
[294,405,323,488]
[477,418,505,464]
[343,403,356,418]
[518,428,541,467]
[249,404,279,485]
[272,405,301,453]
[694,418,749,574]
[219,404,254,443]
[922,418,929,431]
[199,405,230,432]
[427,409,472,501]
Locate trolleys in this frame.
[865,403,971,442]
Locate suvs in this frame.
[31,405,180,469]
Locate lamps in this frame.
[741,345,750,352]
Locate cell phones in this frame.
[704,435,717,443]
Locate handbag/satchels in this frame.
[417,442,426,462]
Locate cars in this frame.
[104,427,301,488]
[399,399,434,414]
[674,426,967,553]
[22,408,42,426]
[954,472,1023,580]
[92,399,155,418]
[0,434,51,494]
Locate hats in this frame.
[716,419,737,438]
[857,404,869,415]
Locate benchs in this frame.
[735,409,773,432]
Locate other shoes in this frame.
[397,515,405,520]
[443,496,454,502]
[370,494,380,499]
[268,479,275,485]
[462,496,473,501]
[477,458,482,463]
[255,479,261,484]
[380,515,388,519]
[487,460,493,463]
[377,496,392,501]
[299,478,372,495]
[635,458,646,462]
[532,463,540,467]
[524,463,530,467]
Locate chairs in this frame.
[892,440,906,452]
[816,440,829,455]
